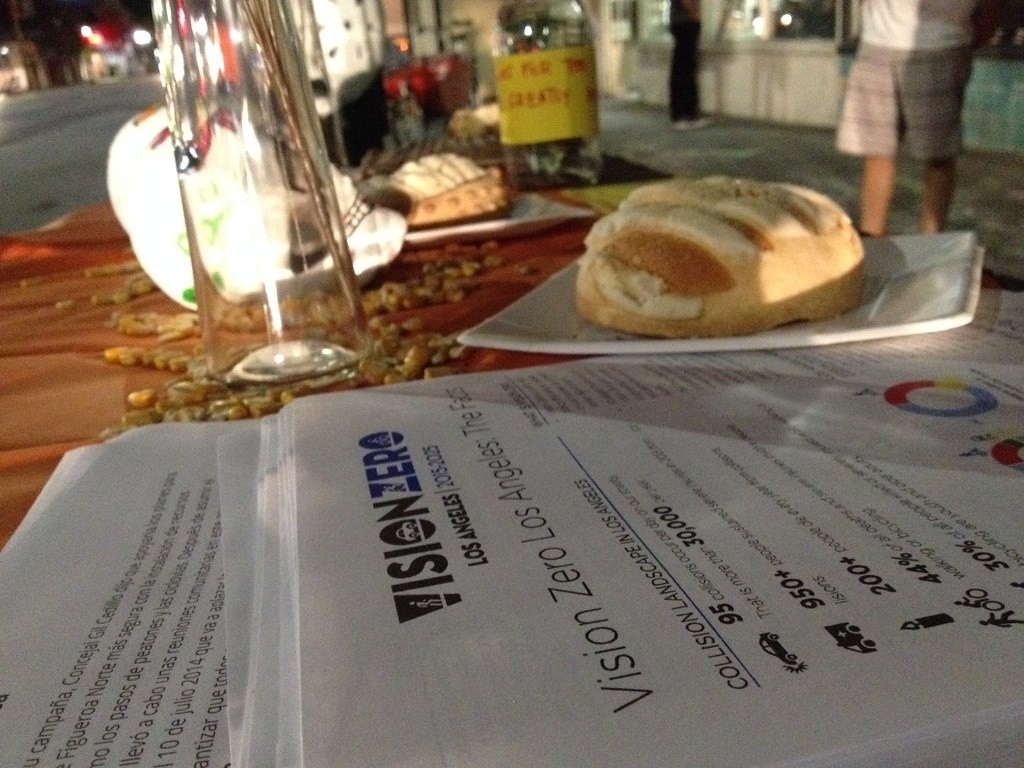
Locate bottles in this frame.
[489,1,601,188]
[150,0,370,387]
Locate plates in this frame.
[405,194,598,252]
[454,228,986,356]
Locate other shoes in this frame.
[674,118,714,129]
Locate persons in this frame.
[832,0,1004,236]
[668,1,714,128]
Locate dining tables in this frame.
[0,153,1008,555]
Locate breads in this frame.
[576,175,866,338]
[383,152,511,231]
